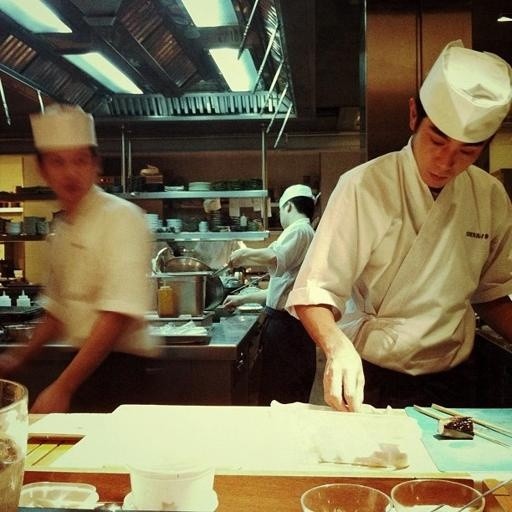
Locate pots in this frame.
[167,256,233,310]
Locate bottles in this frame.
[16,290,32,308]
[157,281,176,318]
[0,290,13,308]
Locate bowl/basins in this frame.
[146,209,221,232]
[6,215,50,236]
[19,480,105,511]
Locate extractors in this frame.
[117,0,204,90]
[97,95,289,121]
[0,24,103,117]
[252,0,291,108]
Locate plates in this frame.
[190,178,263,191]
[28,411,114,439]
[120,494,222,512]
[240,306,263,312]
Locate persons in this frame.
[220,182,318,405]
[0,102,164,413]
[284,36,512,410]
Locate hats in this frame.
[279,184,313,209]
[419,38,511,144]
[30,104,98,147]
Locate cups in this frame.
[240,214,258,231]
[130,438,217,510]
[0,376,29,512]
[301,485,391,511]
[391,479,486,512]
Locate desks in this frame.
[0,406,511,512]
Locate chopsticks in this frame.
[414,402,511,448]
